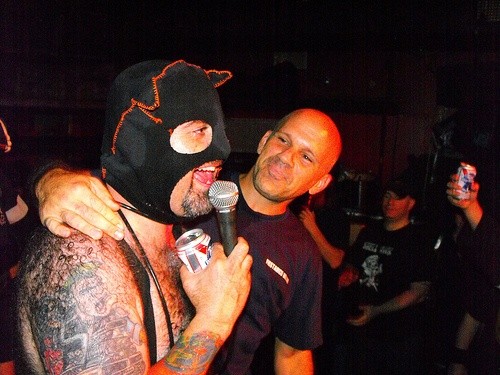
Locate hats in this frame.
[382,177,418,200]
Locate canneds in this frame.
[175,229,214,275]
[453,164,476,200]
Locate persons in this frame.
[13,59,254,375]
[286,187,351,296]
[449,284,500,375]
[322,176,449,375]
[422,111,496,229]
[447,162,500,284]
[0,119,21,375]
[24,108,342,374]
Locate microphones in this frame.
[208,180,239,257]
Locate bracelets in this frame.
[451,346,468,365]
